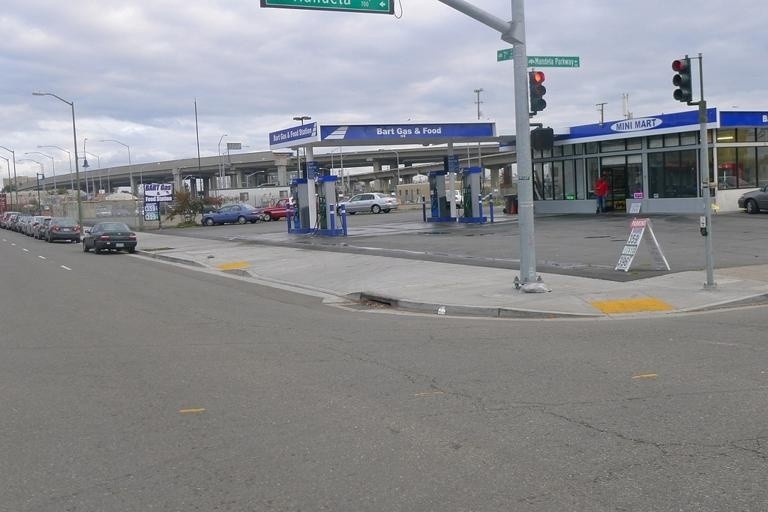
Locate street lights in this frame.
[183,175,193,188]
[331,148,339,176]
[293,116,311,156]
[18,92,99,237]
[247,171,265,187]
[379,149,400,185]
[217,134,228,189]
[102,139,134,194]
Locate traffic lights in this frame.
[528,71,546,111]
[671,58,692,102]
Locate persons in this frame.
[592,174,610,215]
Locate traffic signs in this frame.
[260,0,393,14]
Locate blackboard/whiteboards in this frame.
[614,217,671,271]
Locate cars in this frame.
[337,193,398,216]
[737,185,768,214]
[201,204,265,226]
[0,211,81,243]
[260,198,296,222]
[81,222,137,254]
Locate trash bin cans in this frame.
[503,194,518,214]
